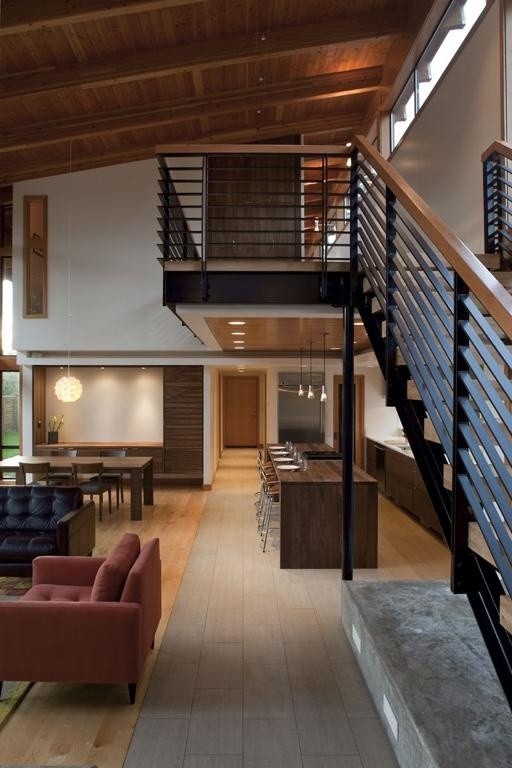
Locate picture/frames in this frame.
[23,194,48,319]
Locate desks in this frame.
[0,454,154,521]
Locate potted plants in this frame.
[48,415,64,444]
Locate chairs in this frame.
[89,448,127,509]
[19,462,62,486]
[39,448,78,480]
[71,462,112,521]
[254,449,280,552]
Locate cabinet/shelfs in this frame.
[35,442,163,473]
[264,442,380,569]
[367,438,443,537]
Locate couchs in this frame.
[0,486,96,577]
[0,532,162,704]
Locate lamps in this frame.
[307,341,315,399]
[314,218,319,232]
[320,331,330,402]
[298,349,304,398]
[54,351,83,403]
